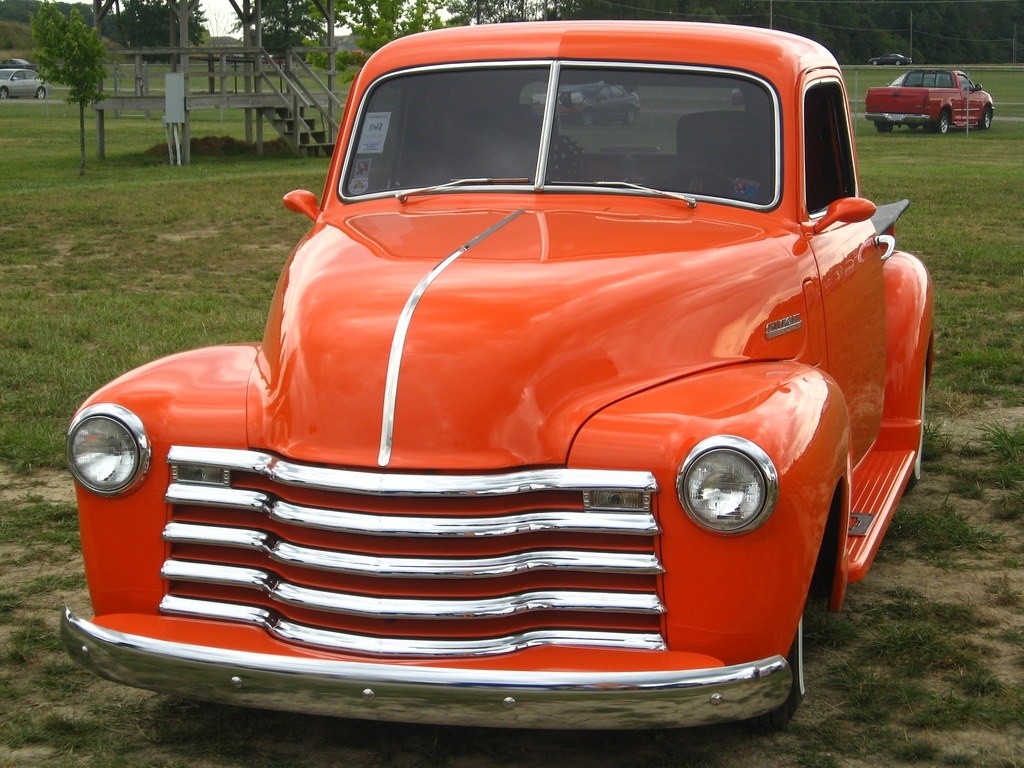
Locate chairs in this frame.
[409,104,559,186]
[676,111,775,205]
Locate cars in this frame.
[867,52,912,66]
[56,16,940,729]
[0,69,48,100]
[0,58,39,72]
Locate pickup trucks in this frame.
[864,67,995,134]
